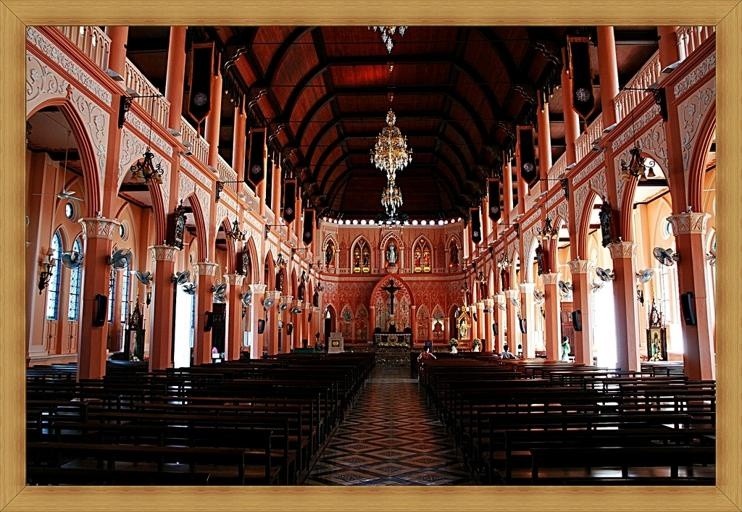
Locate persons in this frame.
[562,336,571,361]
[417,347,437,362]
[314,332,325,352]
[501,346,518,359]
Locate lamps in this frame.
[118,94,164,183]
[621,87,668,179]
[367,26,414,219]
[541,178,569,233]
[216,181,323,292]
[38,248,56,295]
[461,223,519,293]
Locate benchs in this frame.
[419,359,716,487]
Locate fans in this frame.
[595,267,616,283]
[486,279,575,313]
[636,268,655,283]
[171,270,299,314]
[31,130,84,203]
[55,248,86,269]
[136,271,154,285]
[106,248,133,269]
[653,247,681,267]
